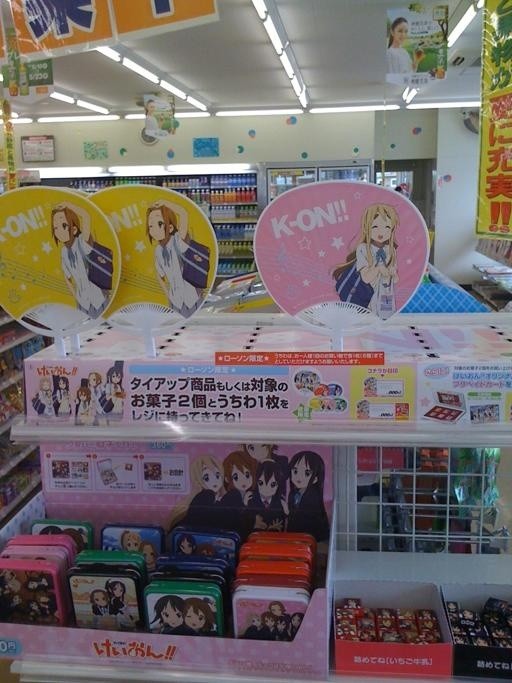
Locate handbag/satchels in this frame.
[88,243,113,290]
[334,266,374,309]
[183,240,210,288]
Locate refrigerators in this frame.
[267,165,370,207]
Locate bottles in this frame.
[211,173,258,275]
[69,177,211,206]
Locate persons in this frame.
[144,98,165,132]
[387,16,425,76]
